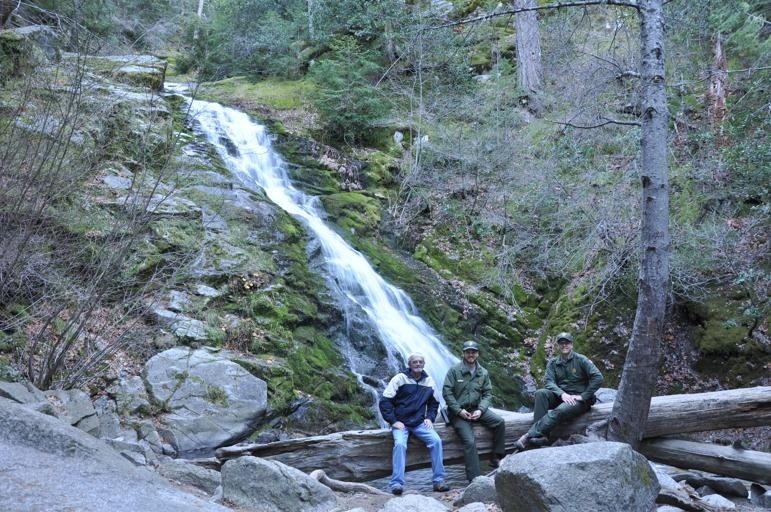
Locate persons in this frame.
[379,352,449,495]
[514,330,605,451]
[442,340,506,483]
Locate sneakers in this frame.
[433,481,449,491]
[489,452,500,464]
[392,485,403,495]
[516,432,549,451]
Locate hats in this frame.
[462,341,479,350]
[556,332,572,342]
